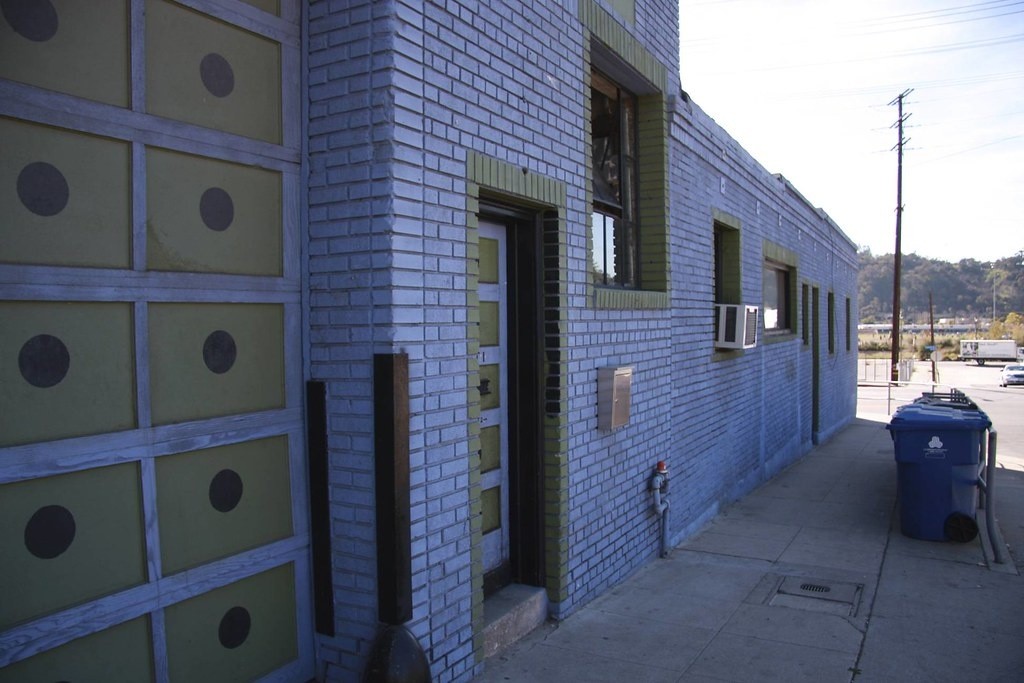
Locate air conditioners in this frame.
[714,303,760,349]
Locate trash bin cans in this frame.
[885,395,991,544]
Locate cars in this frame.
[999,363,1024,387]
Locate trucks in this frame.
[958,340,1024,365]
